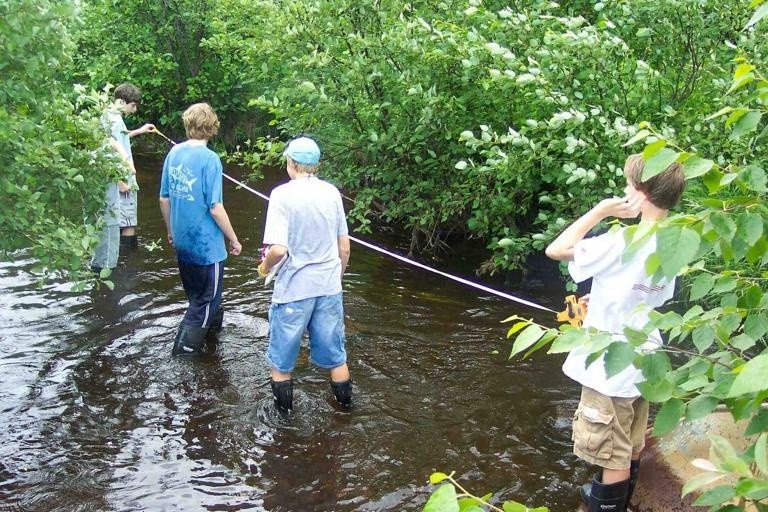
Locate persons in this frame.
[77,132,136,276]
[158,102,244,357]
[100,83,156,246]
[546,150,685,510]
[257,135,353,411]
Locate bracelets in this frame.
[257,264,270,277]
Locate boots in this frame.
[578,454,641,512]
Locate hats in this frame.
[283,137,321,165]
